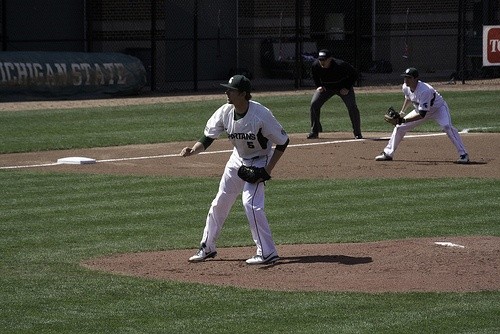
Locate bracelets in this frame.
[405,119,406,124]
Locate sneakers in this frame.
[188,247,217,262]
[245,250,280,265]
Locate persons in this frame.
[181,75,290,264]
[375,68,471,164]
[307,49,362,139]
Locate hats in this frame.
[318,49,331,60]
[400,67,420,78]
[220,74,252,92]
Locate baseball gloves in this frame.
[384,106,405,126]
[237,166,271,185]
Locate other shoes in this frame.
[307,132,319,139]
[375,152,392,161]
[354,134,362,139]
[456,153,470,162]
